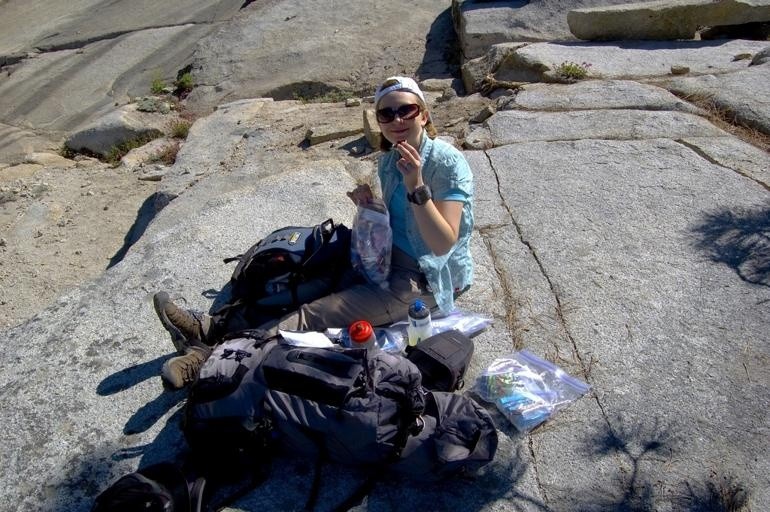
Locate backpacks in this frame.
[230,223,360,308]
[194,328,500,479]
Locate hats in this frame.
[373,75,425,106]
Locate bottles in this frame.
[408,298,432,347]
[350,320,381,357]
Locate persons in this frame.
[152,74,476,392]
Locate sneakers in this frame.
[153,291,211,354]
[160,346,212,390]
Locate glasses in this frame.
[376,105,419,123]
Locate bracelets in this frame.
[407,184,433,206]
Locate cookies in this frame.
[391,139,408,150]
